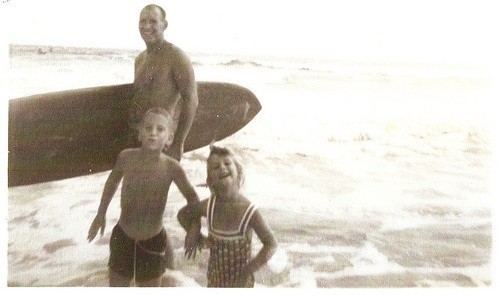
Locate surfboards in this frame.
[9,83,261,186]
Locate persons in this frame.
[177,145,277,288]
[129,4,198,271]
[88,108,201,288]
